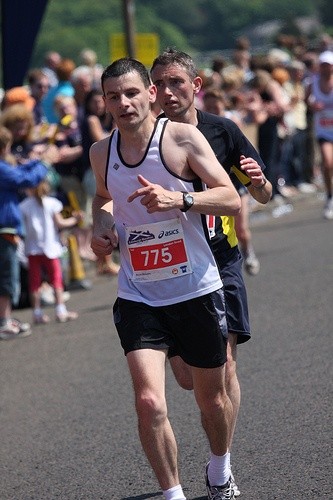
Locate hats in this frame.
[5,87,36,110]
[318,51,333,66]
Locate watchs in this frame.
[180,191,193,212]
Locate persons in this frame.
[90,57,241,500]
[0,32,333,339]
[150,47,272,496]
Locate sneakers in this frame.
[204,461,241,500]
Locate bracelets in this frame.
[254,178,267,189]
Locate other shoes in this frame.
[68,279,93,288]
[55,311,78,322]
[240,244,261,275]
[0,318,32,338]
[39,283,71,305]
[33,313,49,324]
[323,199,333,219]
